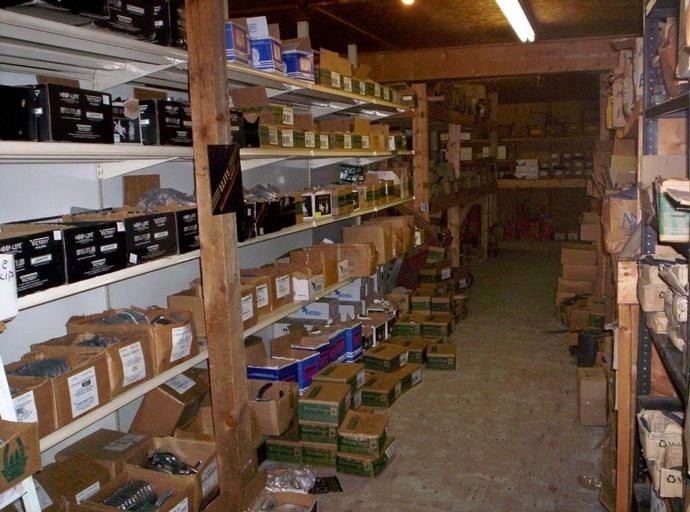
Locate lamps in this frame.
[495,0,538,43]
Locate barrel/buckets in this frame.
[577,327,603,367]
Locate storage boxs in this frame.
[498,106,599,250]
